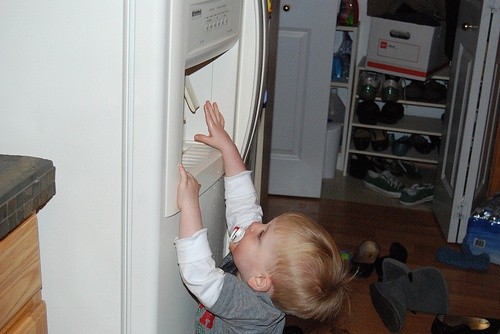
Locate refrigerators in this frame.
[0,0,269,333]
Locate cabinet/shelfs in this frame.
[331,21,483,176]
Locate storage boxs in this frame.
[365,0,450,82]
[322,121,344,179]
[466,217,500,265]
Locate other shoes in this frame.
[358,102,404,125]
[363,170,402,198]
[359,72,403,102]
[350,239,380,277]
[353,128,388,151]
[400,80,446,103]
[436,244,490,271]
[350,155,389,177]
[393,135,430,156]
[431,313,500,334]
[400,184,434,206]
[389,159,421,179]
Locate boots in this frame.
[371,258,448,331]
